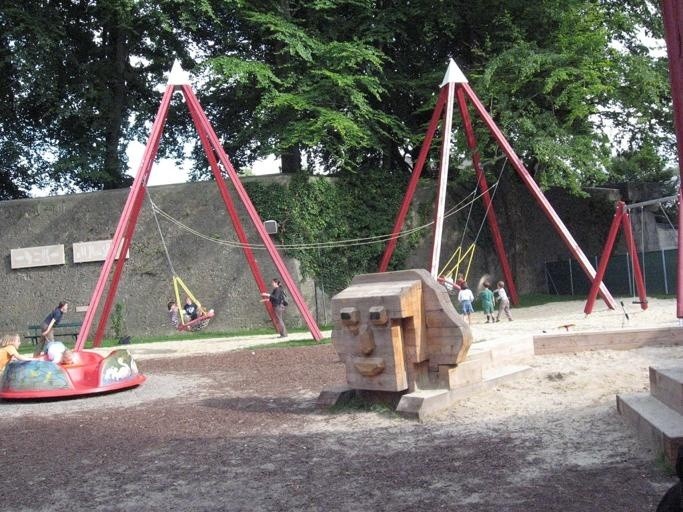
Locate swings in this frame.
[145,189,215,332]
[438,157,508,295]
[658,199,678,232]
[629,206,648,304]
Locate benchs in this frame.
[24,322,85,347]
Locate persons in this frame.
[444,270,514,326]
[259,278,288,338]
[33,301,68,358]
[0,332,46,376]
[183,297,208,320]
[168,302,182,330]
